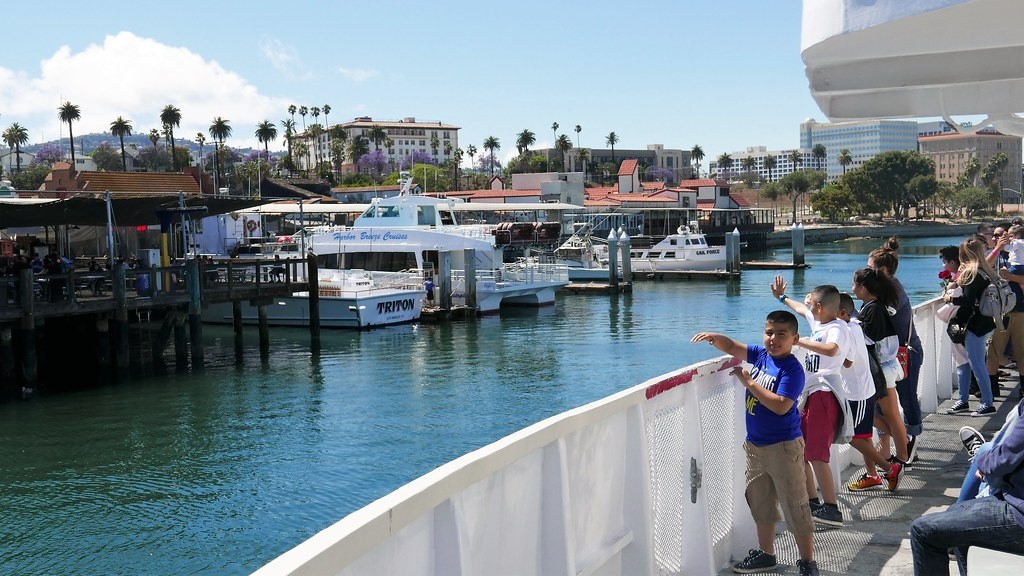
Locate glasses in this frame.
[992,233,1002,239]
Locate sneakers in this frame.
[876,457,912,471]
[881,464,905,491]
[959,426,986,464]
[808,501,843,527]
[912,451,918,462]
[875,455,895,468]
[947,400,969,413]
[971,402,996,416]
[733,549,777,573]
[905,434,919,464]
[848,473,883,492]
[796,559,819,576]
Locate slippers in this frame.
[997,370,1011,377]
[996,376,1005,382]
[998,361,1017,369]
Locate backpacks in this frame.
[977,268,1016,317]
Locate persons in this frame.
[118,256,136,288]
[423,278,435,307]
[938,218,1024,416]
[852,268,917,473]
[769,274,848,525]
[87,257,111,296]
[691,311,819,576]
[196,255,217,288]
[227,241,241,257]
[6,253,49,305]
[911,398,1024,575]
[804,293,904,493]
[268,255,284,283]
[866,236,924,465]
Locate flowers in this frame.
[938,269,952,289]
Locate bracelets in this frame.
[779,295,786,302]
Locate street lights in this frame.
[1015,181,1022,216]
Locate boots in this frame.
[976,373,999,397]
[1019,374,1024,399]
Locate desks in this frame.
[269,264,283,284]
[205,269,218,282]
[252,265,267,282]
[79,275,106,296]
[34,279,50,293]
[218,268,227,282]
[232,266,251,283]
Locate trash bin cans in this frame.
[136,272,150,296]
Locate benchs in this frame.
[207,272,289,286]
[34,278,136,302]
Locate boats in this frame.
[246,147,570,312]
[549,196,726,280]
[201,232,429,329]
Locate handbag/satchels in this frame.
[896,346,909,379]
[946,318,966,344]
